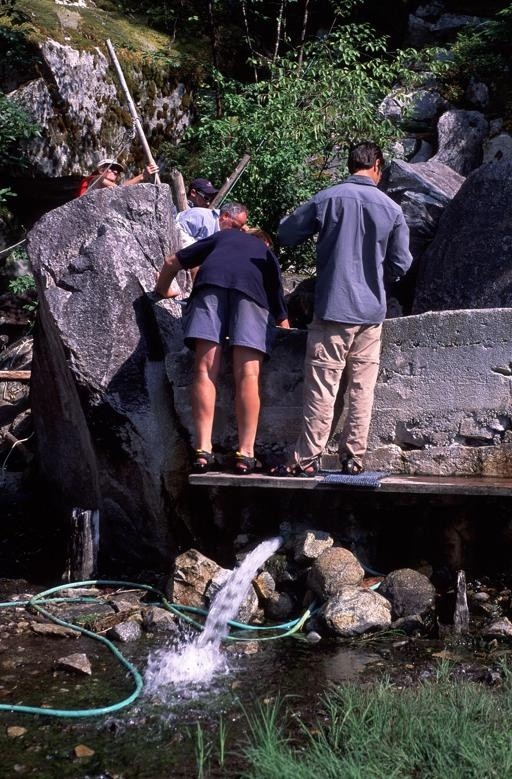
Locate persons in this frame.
[174,202,247,283]
[154,230,290,475]
[79,157,160,196]
[186,178,219,208]
[278,141,414,478]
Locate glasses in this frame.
[195,190,216,200]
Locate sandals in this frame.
[266,459,320,477]
[341,454,366,475]
[233,451,263,475]
[192,447,221,473]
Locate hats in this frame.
[96,158,125,172]
[189,178,219,194]
